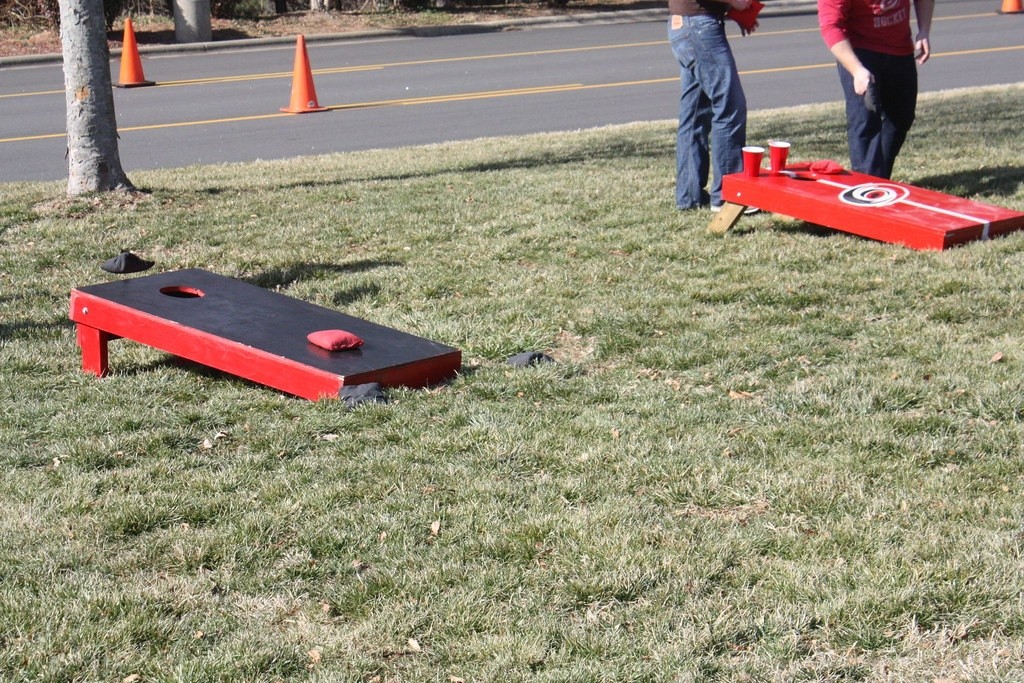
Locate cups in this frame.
[768,141,791,174]
[742,146,765,177]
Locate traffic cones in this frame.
[997,0,1024,15]
[278,34,329,112]
[111,17,156,88]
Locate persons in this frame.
[817,0,935,179]
[668,0,759,214]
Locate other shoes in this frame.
[708,199,762,215]
